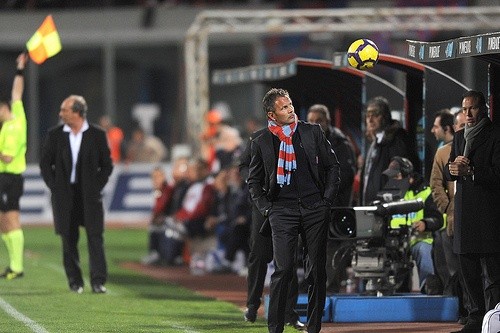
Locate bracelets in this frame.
[14,68,24,76]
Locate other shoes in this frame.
[92,284,108,294]
[70,285,84,294]
[0,268,24,279]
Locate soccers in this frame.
[347,38,380,71]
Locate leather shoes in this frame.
[285,320,306,331]
[244,307,257,324]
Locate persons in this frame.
[0,53,27,280]
[40,94,108,292]
[98,88,500,333]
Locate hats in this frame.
[382,158,414,177]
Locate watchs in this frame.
[467,165,474,177]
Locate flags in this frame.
[26,14,63,64]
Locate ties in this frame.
[363,137,378,191]
